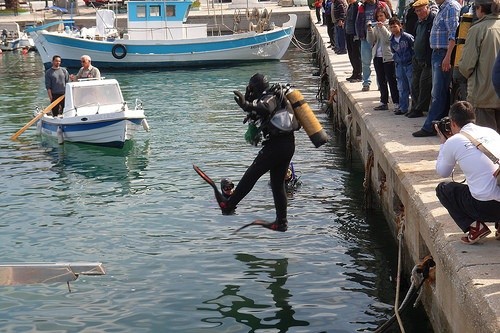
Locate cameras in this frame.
[369,23,377,28]
[431,117,450,133]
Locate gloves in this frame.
[233,90,249,110]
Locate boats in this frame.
[0,22,38,51]
[22,0,297,72]
[33,79,148,150]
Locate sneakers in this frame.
[496,228,500,240]
[461,220,491,245]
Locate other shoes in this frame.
[332,47,338,51]
[412,128,438,137]
[405,109,425,118]
[373,104,391,110]
[335,49,347,54]
[362,85,369,90]
[393,103,400,112]
[346,75,363,83]
[327,41,332,44]
[395,110,406,115]
[327,44,335,48]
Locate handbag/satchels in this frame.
[493,164,500,187]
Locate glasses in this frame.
[223,183,234,191]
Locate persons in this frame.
[367,5,399,111]
[459,0,500,132]
[45,56,69,116]
[322,0,348,54]
[434,101,500,244]
[405,0,435,118]
[220,178,235,198]
[194,73,296,232]
[346,0,362,82]
[314,0,322,24]
[70,55,100,81]
[285,169,293,185]
[355,0,392,91]
[389,16,415,115]
[412,0,462,136]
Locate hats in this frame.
[221,178,231,189]
[412,0,429,7]
